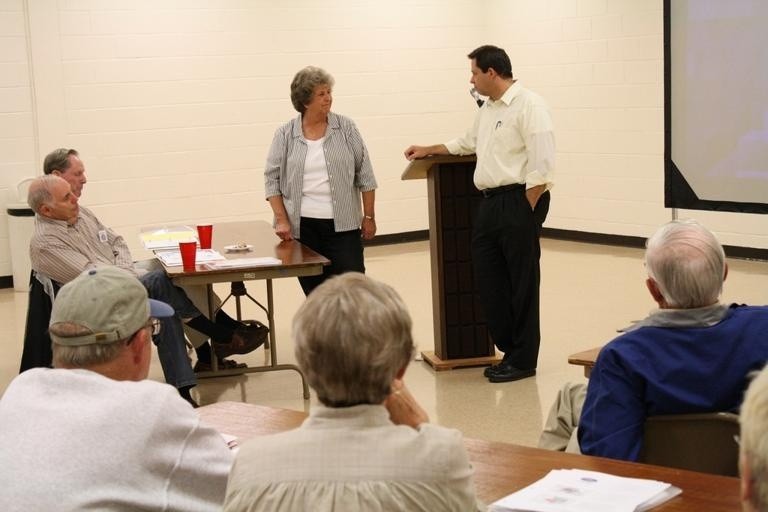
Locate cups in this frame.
[197,225,213,249]
[179,242,197,270]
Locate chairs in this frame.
[637,413,740,479]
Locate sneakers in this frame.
[192,359,249,380]
[212,319,269,359]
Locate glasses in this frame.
[126,318,162,346]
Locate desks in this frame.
[567,346,603,377]
[192,400,743,512]
[141,221,333,400]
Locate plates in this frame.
[226,251,253,255]
[224,243,252,251]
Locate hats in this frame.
[47,265,174,349]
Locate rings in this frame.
[393,390,403,396]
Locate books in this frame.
[139,224,225,268]
[487,465,685,512]
[218,430,240,449]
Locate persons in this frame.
[536,220,768,461]
[263,62,379,301]
[404,45,556,383]
[30,147,259,380]
[224,271,486,512]
[734,363,768,510]
[28,174,268,410]
[1,263,235,512]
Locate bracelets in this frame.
[363,214,376,223]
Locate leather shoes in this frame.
[482,360,537,383]
